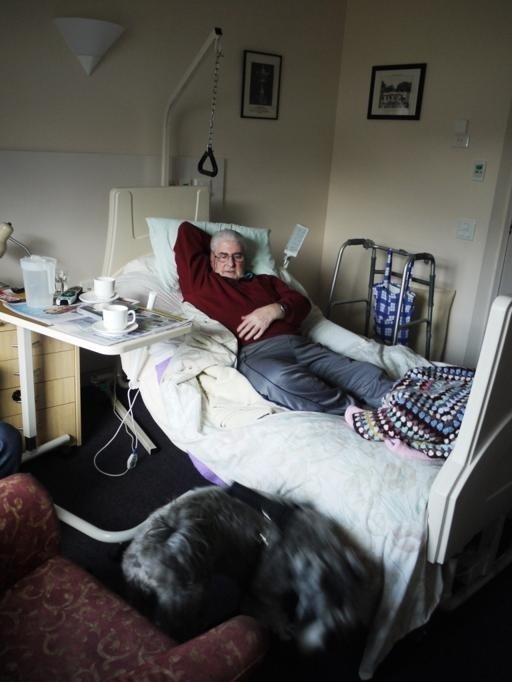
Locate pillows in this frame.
[146,218,280,287]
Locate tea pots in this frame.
[20,254,58,309]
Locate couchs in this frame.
[1,473,264,680]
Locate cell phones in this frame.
[55,287,82,306]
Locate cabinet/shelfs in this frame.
[5,298,82,448]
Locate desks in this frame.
[1,291,193,465]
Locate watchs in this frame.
[274,299,290,320]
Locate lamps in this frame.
[0,220,32,259]
[54,16,125,76]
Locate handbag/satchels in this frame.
[373,249,416,347]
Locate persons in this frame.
[0,420,24,477]
[173,221,400,416]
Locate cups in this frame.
[94,276,115,299]
[101,304,137,333]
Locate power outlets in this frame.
[451,136,469,147]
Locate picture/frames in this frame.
[242,50,283,120]
[367,63,426,120]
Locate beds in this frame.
[103,186,512,680]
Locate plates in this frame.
[79,290,121,304]
[91,320,139,337]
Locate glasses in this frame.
[211,250,246,263]
